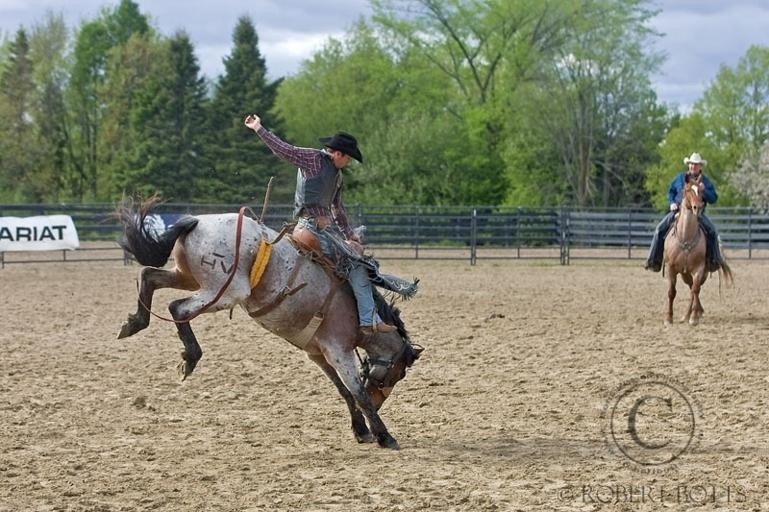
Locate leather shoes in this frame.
[362,322,397,335]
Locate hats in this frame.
[683,152,707,167]
[319,130,363,163]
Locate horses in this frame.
[657,179,735,327]
[110,189,425,452]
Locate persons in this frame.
[244,114,397,335]
[645,152,722,272]
